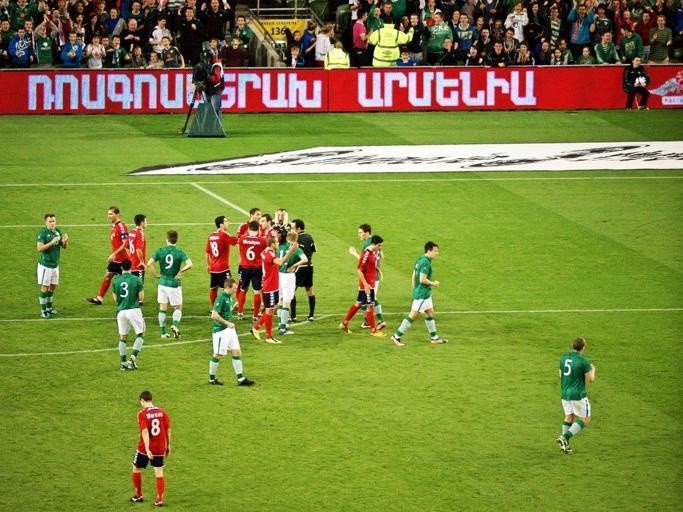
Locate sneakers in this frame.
[237,377,254,386]
[556,435,569,451]
[339,320,448,345]
[160,333,170,338]
[170,325,179,338]
[40,310,49,318]
[208,378,223,385]
[119,362,127,370]
[129,495,143,502]
[127,361,137,369]
[208,300,313,344]
[86,296,101,305]
[46,308,56,314]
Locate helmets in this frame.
[201,50,212,58]
[635,74,647,88]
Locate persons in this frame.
[555,338,595,454]
[130,390,172,506]
[390,242,448,347]
[197,47,225,122]
[36,214,69,318]
[622,56,650,111]
